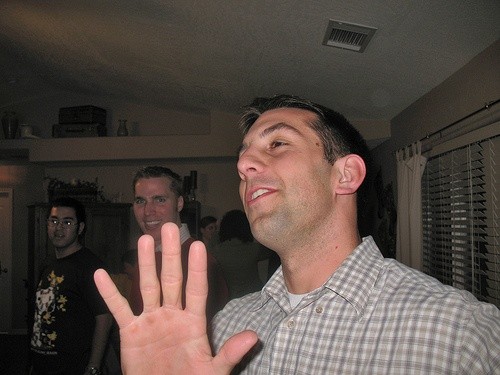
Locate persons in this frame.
[125,166,228,322]
[26,197,113,375]
[94,95,500,375]
[207,209,255,272]
[199,216,218,246]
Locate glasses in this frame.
[47,217,78,227]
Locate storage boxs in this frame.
[52,105,108,138]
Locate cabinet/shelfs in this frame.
[29,202,200,343]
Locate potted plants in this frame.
[43,175,111,214]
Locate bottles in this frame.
[116,120,129,137]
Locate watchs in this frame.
[85,366,102,375]
